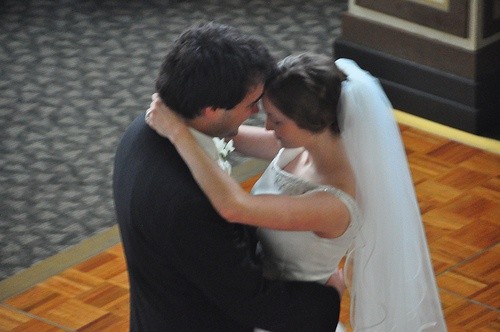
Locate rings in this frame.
[146,110,152,117]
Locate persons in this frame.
[112,23,346,332]
[144,52,366,332]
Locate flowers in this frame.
[212,137,235,177]
